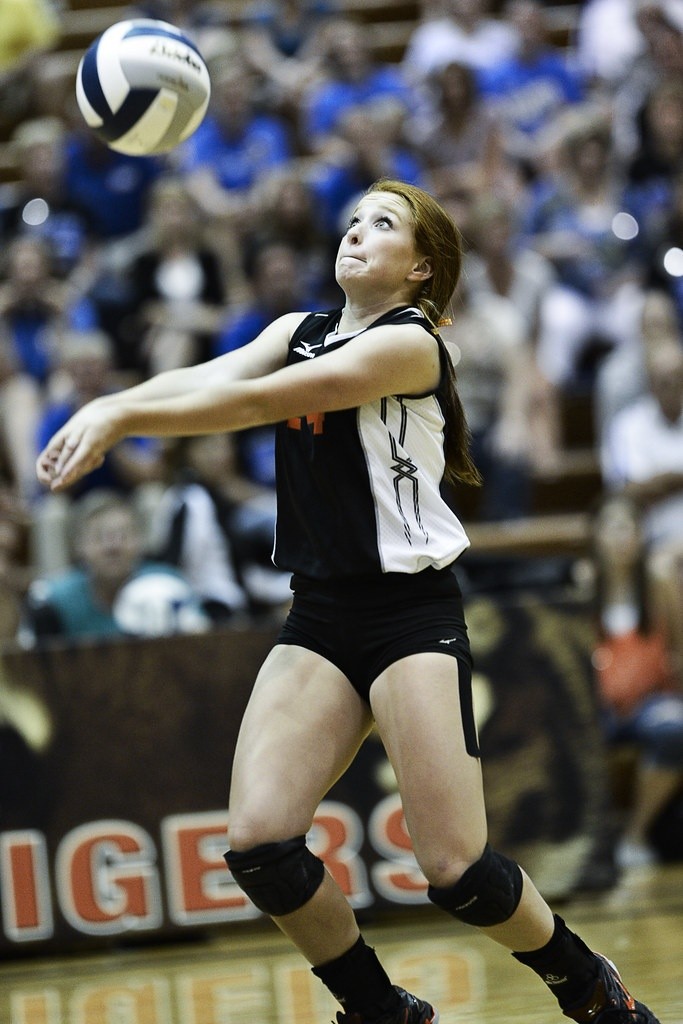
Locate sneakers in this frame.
[330,984,439,1024]
[558,951,663,1024]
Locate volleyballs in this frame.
[73,16,213,159]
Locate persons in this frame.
[0,0,681,910]
[35,182,656,1024]
